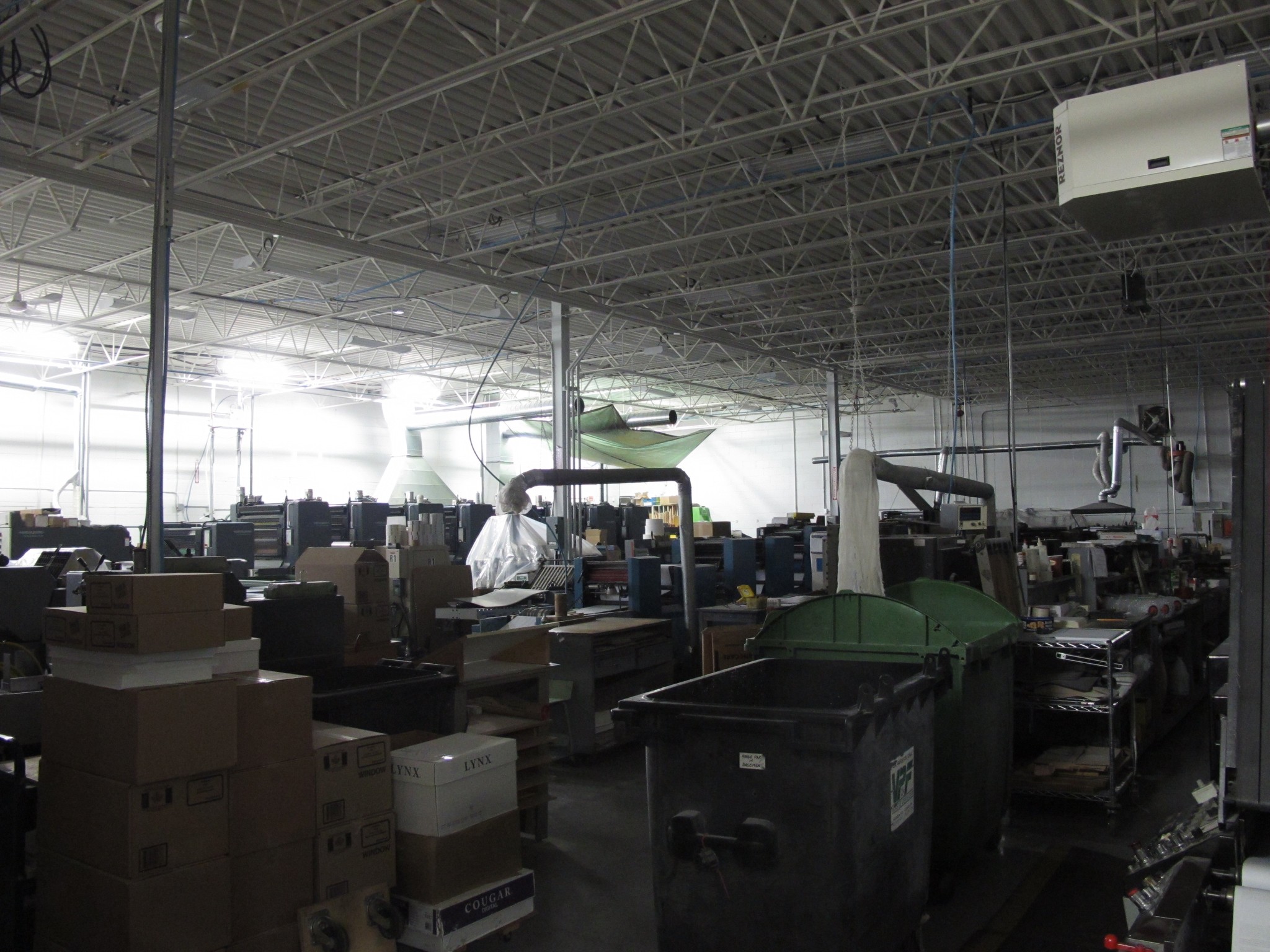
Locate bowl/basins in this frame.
[1048,555,1064,572]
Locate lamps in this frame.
[508,366,554,378]
[232,236,336,286]
[83,83,220,147]
[632,331,797,411]
[479,291,552,333]
[99,284,198,323]
[741,130,898,178]
[329,325,412,355]
[429,204,566,245]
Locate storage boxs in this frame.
[31,542,535,952]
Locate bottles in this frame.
[1023,538,1052,582]
[476,491,480,504]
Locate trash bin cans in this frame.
[744,571,1024,904]
[609,655,955,952]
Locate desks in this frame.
[695,592,806,644]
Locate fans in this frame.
[798,249,904,319]
[1,253,63,317]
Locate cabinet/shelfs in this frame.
[546,615,675,759]
[441,658,564,846]
